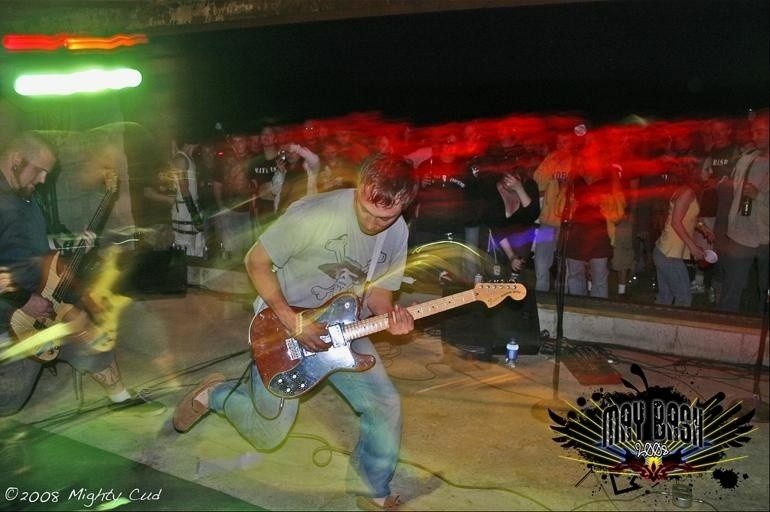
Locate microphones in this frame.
[575,124,586,152]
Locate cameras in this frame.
[279,149,286,161]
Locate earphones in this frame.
[13,164,17,173]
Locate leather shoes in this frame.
[356,494,412,512]
[173,373,227,433]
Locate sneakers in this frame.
[105,386,162,410]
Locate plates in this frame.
[704,249,719,264]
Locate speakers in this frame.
[120,251,188,300]
[441,283,540,354]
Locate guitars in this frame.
[9,166,119,363]
[249,280,527,398]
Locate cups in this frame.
[672,485,693,508]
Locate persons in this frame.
[172,153,419,511]
[0,129,167,418]
[419,111,770,362]
[140,125,320,263]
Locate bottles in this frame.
[510,257,521,281]
[741,183,753,216]
[505,336,520,370]
[168,240,225,261]
